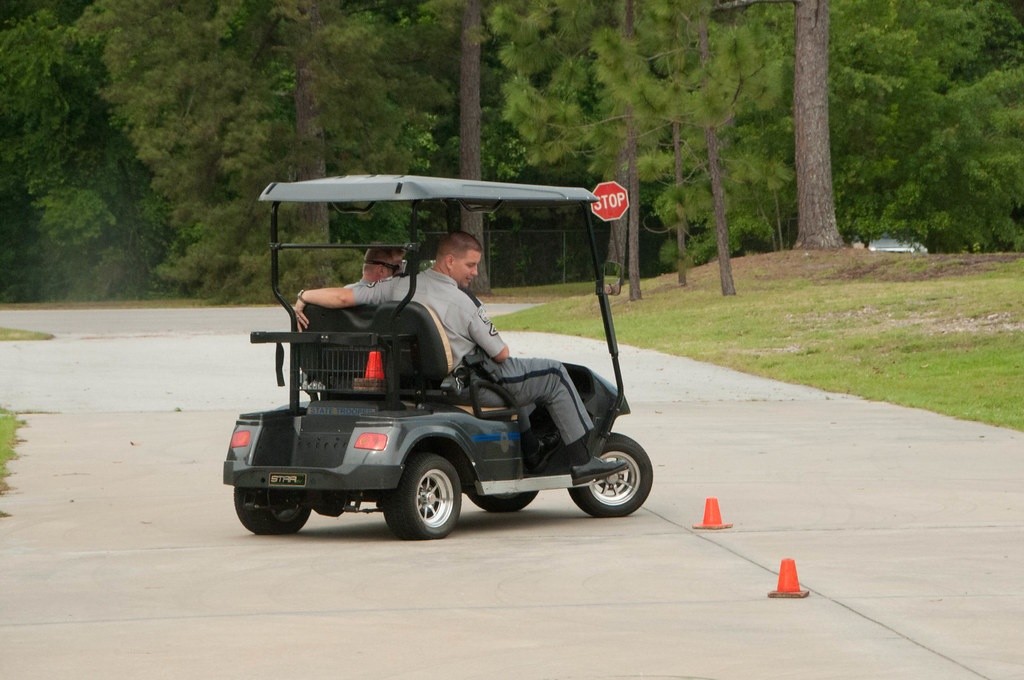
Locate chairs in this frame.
[367,299,523,420]
[295,304,369,397]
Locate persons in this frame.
[340,243,408,290]
[293,229,629,487]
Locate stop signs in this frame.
[591,180,628,221]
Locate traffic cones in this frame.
[691,497,732,530]
[767,558,809,599]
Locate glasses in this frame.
[368,260,399,274]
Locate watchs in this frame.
[297,289,307,304]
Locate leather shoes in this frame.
[525,431,562,473]
[571,454,629,486]
